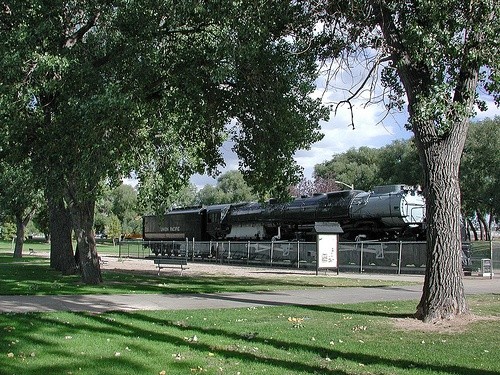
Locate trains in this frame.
[142,184,473,270]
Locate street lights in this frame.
[335,181,355,190]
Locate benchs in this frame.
[29,248,37,255]
[154,259,190,276]
[99,257,109,270]
